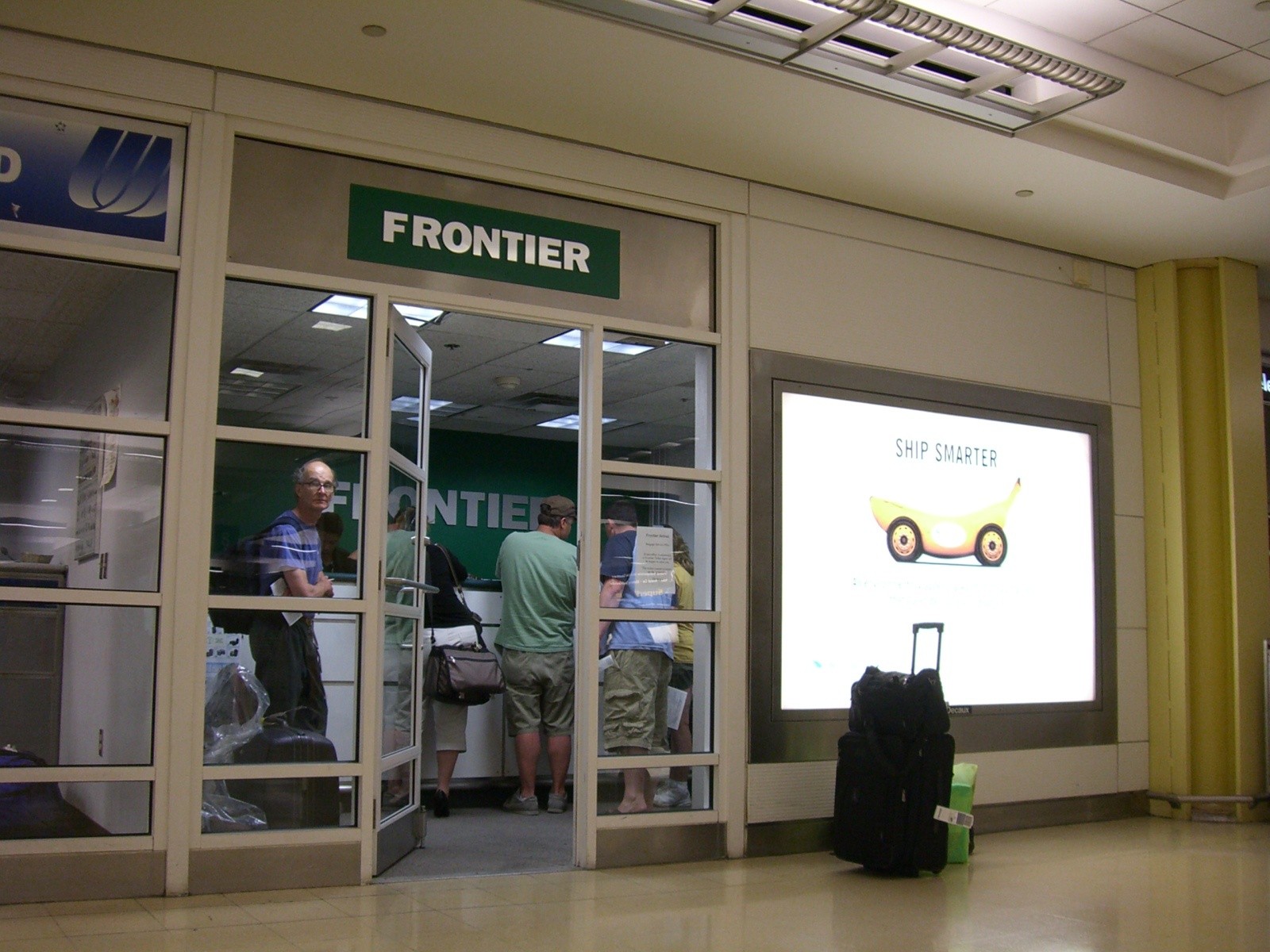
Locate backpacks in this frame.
[209,516,305,633]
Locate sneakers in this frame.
[656,778,671,791]
[654,782,690,806]
[546,791,569,812]
[503,788,539,815]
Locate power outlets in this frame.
[99,729,103,758]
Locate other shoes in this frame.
[380,790,410,812]
[433,790,450,818]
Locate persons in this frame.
[316,512,349,574]
[653,525,694,806]
[494,496,577,816]
[382,507,413,792]
[599,499,678,815]
[249,457,339,736]
[382,542,483,817]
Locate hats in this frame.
[540,495,577,520]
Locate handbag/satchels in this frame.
[423,645,506,707]
[848,665,949,738]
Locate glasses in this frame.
[300,481,336,494]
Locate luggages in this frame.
[227,716,340,830]
[828,622,953,877]
